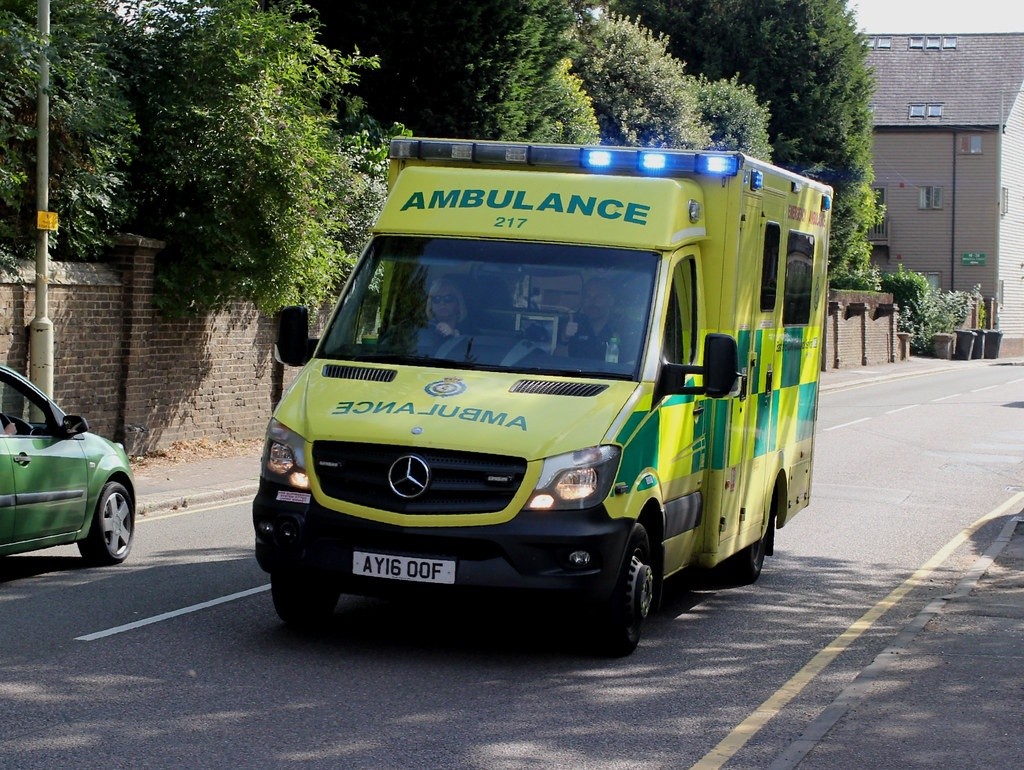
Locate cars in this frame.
[0,363,135,568]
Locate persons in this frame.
[0,420,17,435]
[423,279,468,338]
[558,277,643,366]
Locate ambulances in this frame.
[252,135,834,656]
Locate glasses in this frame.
[431,294,458,304]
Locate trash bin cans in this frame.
[953,330,978,361]
[971,328,988,360]
[985,328,1004,359]
